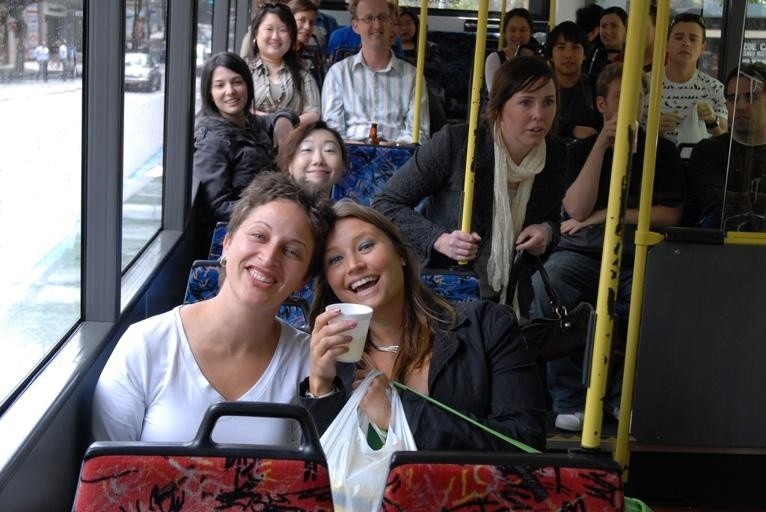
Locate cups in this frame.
[324,303,374,363]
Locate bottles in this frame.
[366,123,378,145]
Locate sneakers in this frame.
[555,412,584,432]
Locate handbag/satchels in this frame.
[506,249,595,359]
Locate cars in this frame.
[193,22,234,72]
[122,53,161,92]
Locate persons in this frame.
[545,61,686,433]
[32,36,77,83]
[294,198,547,454]
[373,56,565,320]
[89,1,764,232]
[92,171,335,457]
